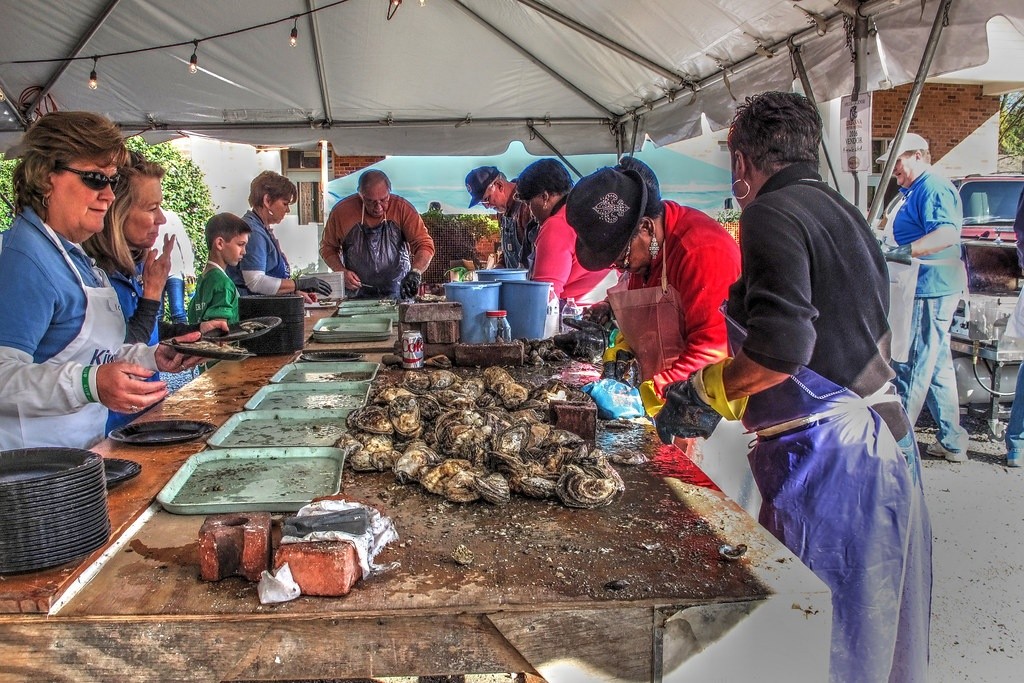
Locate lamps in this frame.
[188,54,198,75]
[89,70,98,90]
[415,0,429,7]
[288,26,299,48]
[0,89,6,102]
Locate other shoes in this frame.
[1006,449,1024,466]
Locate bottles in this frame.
[484,310,511,344]
[547,287,559,336]
[582,307,587,320]
[562,298,581,334]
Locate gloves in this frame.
[293,277,332,296]
[888,243,912,256]
[655,355,750,445]
[602,331,638,381]
[639,380,666,419]
[555,316,611,362]
[400,272,420,299]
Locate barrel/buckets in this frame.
[477,268,529,283]
[495,279,553,339]
[443,281,502,345]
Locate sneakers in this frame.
[925,440,968,462]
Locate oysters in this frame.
[334,366,627,510]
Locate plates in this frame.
[160,341,257,361]
[300,352,363,361]
[238,293,305,354]
[202,315,282,343]
[0,447,112,575]
[242,314,242,316]
[103,458,142,487]
[108,420,217,445]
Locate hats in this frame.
[466,165,499,209]
[565,167,646,272]
[875,134,929,164]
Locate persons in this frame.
[560,153,738,405]
[651,89,941,683]
[511,155,622,307]
[229,170,331,305]
[0,109,212,453]
[428,201,443,215]
[93,145,230,440]
[462,165,541,271]
[145,205,196,349]
[872,127,970,464]
[185,211,251,331]
[318,172,436,298]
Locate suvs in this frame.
[953,175,1024,245]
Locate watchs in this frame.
[996,184,1024,470]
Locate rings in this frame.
[129,403,139,413]
[180,364,187,372]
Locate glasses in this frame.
[53,163,120,192]
[606,237,632,272]
[478,197,490,205]
[360,194,389,206]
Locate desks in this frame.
[0,296,835,683]
